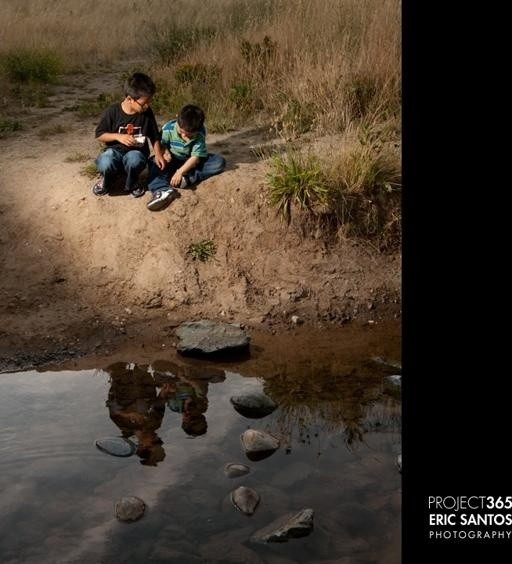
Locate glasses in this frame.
[132,98,155,108]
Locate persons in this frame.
[92,72,167,196]
[147,103,227,212]
[154,372,209,437]
[105,364,167,466]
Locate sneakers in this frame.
[131,182,144,197]
[146,188,175,211]
[92,173,111,195]
